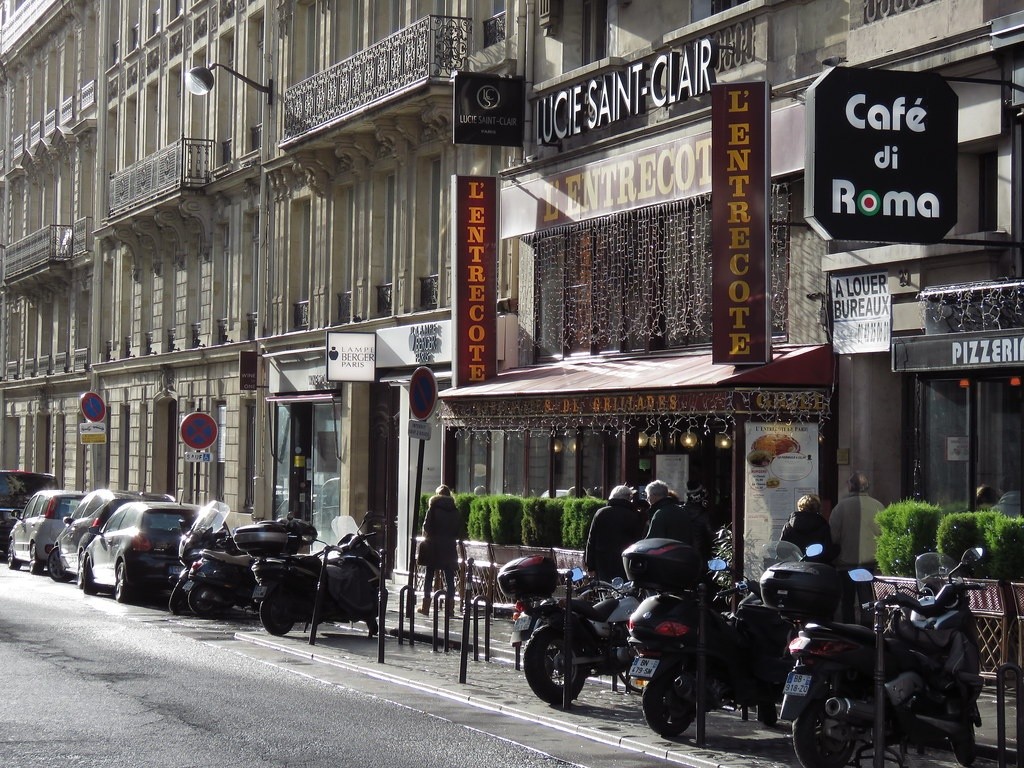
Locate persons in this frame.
[567,487,589,499]
[417,484,461,619]
[776,494,834,564]
[474,485,487,495]
[975,477,1021,516]
[582,480,722,585]
[828,471,886,631]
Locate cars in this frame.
[82,501,200,602]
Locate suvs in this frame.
[7,489,90,574]
[47,489,177,587]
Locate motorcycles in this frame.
[168,525,249,614]
[620,538,824,736]
[497,553,656,705]
[760,545,985,768]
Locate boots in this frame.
[449,600,455,617]
[417,597,432,615]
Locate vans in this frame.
[0,470,59,552]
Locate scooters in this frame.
[232,511,380,638]
[182,548,255,616]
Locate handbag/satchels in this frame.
[418,542,432,566]
